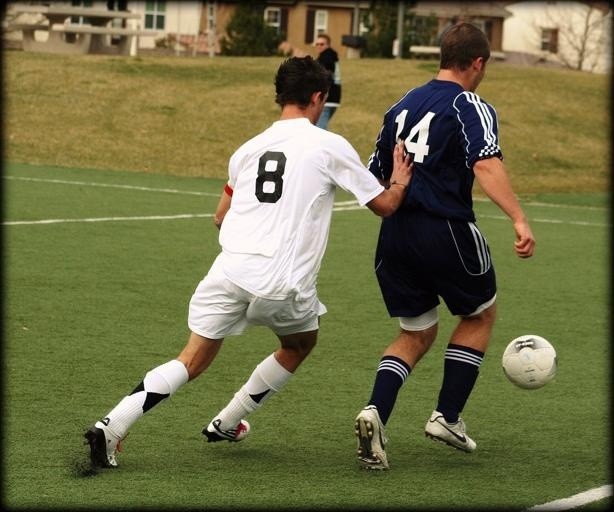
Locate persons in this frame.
[351,19,539,472]
[81,54,416,471]
[314,33,342,131]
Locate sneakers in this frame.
[83,418,121,469]
[423,408,476,452]
[353,404,389,470]
[201,419,250,443]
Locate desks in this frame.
[14,7,141,57]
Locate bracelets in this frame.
[391,180,408,190]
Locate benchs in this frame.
[14,21,47,53]
[51,22,159,58]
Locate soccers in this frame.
[502,335,558,389]
